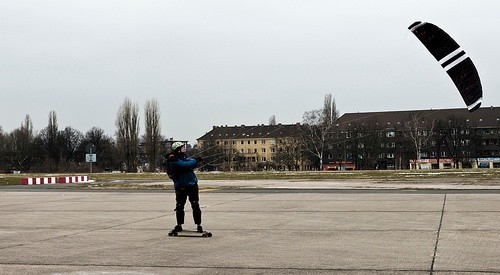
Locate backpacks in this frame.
[166,155,174,179]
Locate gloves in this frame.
[194,156,203,163]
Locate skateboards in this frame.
[168,228,213,238]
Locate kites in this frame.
[407,19,484,115]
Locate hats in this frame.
[172,142,185,150]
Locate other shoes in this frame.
[172,225,182,232]
[197,226,204,233]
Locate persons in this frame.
[166,141,203,234]
